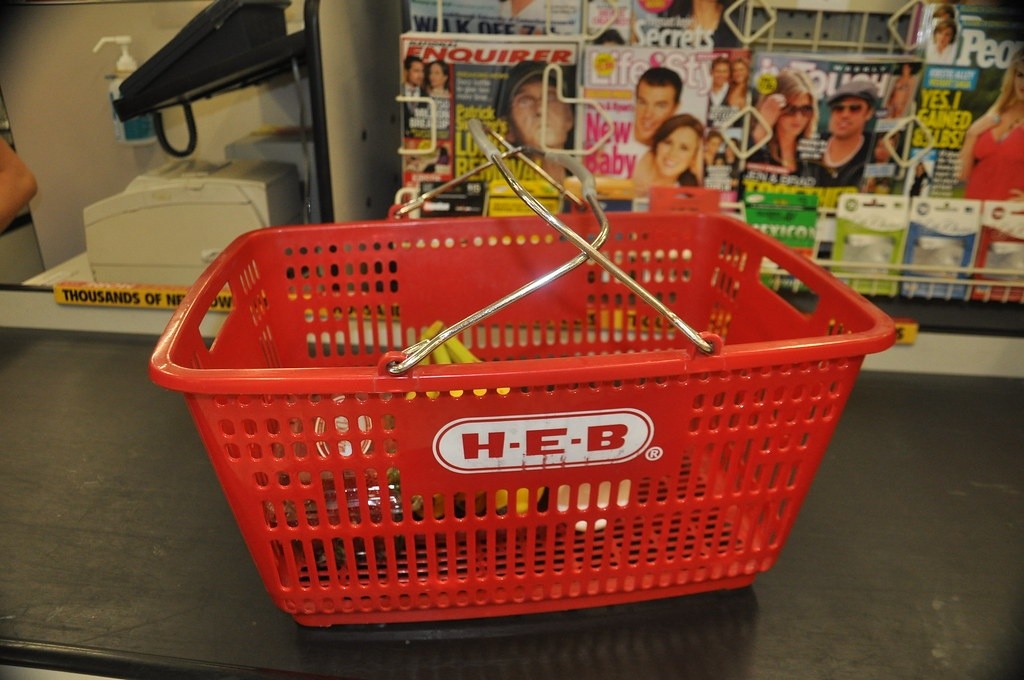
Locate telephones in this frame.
[113,1,286,118]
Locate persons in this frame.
[708,56,750,124]
[875,64,914,163]
[933,7,956,60]
[635,68,701,195]
[500,59,574,177]
[749,73,878,186]
[704,132,739,189]
[1,137,39,233]
[403,56,451,131]
[958,50,1024,270]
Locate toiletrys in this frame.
[92,35,159,148]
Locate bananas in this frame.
[400,321,549,516]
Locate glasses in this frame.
[783,104,813,118]
[832,102,863,111]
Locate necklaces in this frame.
[1000,117,1021,132]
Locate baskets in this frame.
[147,119,897,627]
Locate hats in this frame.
[828,80,878,105]
[492,60,569,118]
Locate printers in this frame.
[87,149,303,289]
